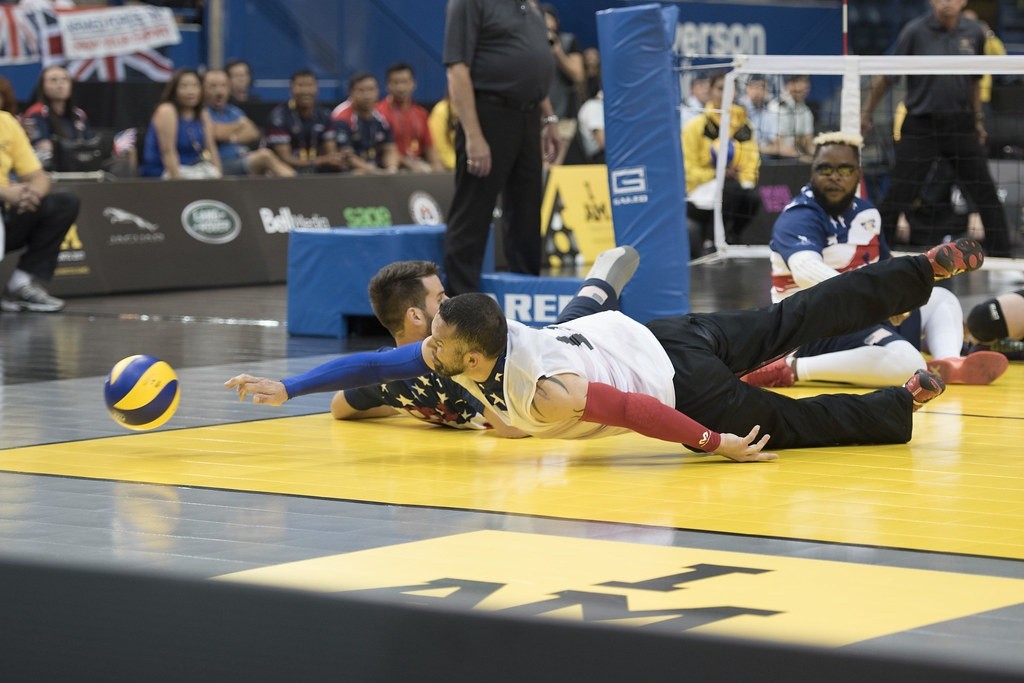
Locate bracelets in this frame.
[542,115,558,125]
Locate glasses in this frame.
[815,163,856,178]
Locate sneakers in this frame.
[928,350,1009,385]
[903,369,945,412]
[926,237,984,281]
[1,277,66,313]
[992,338,1024,359]
[741,354,795,388]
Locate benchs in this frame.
[286,224,497,338]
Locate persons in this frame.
[332,246,640,439]
[0,0,1009,311]
[224,236,986,461]
[966,291,1024,358]
[741,131,1008,390]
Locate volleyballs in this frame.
[104,353,181,431]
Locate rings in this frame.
[467,160,472,165]
